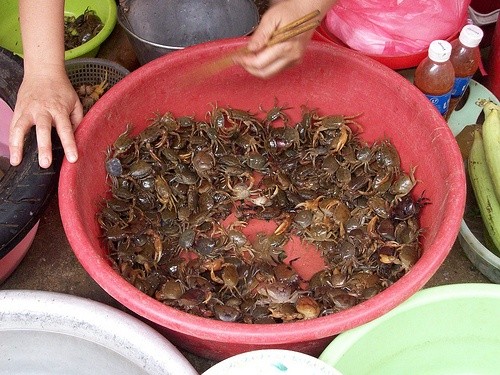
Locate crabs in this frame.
[71,69,109,114]
[64,5,105,52]
[93,92,432,325]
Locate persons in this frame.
[8,0,338,169]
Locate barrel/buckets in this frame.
[116,1,265,69]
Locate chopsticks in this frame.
[180,9,322,86]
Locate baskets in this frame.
[64,58,129,111]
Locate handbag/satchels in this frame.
[324,0,470,57]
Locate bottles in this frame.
[414,39,455,120]
[446,24,483,122]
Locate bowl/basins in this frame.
[317,283,500,375]
[0,98,42,287]
[58,35,467,362]
[0,289,195,375]
[446,79,500,283]
[313,13,468,70]
[0,0,118,73]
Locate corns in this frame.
[468,98,500,252]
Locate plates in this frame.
[200,348,342,375]
